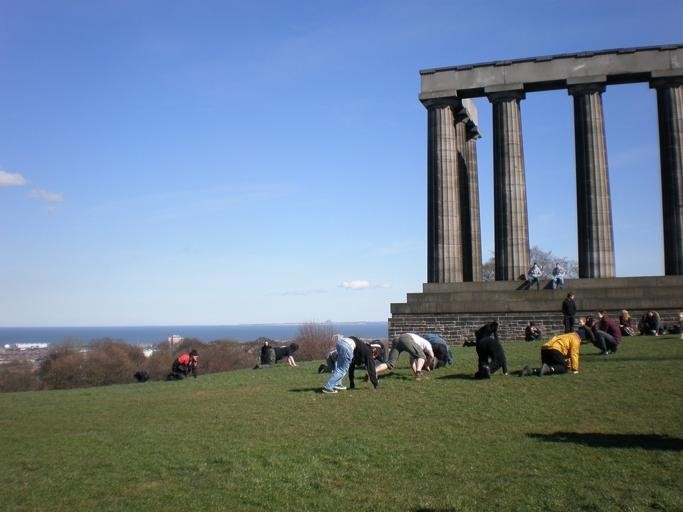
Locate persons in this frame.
[526,263,542,291]
[520,322,585,377]
[319,332,453,394]
[463,322,508,379]
[562,293,577,333]
[164,350,199,381]
[551,262,566,290]
[618,310,683,336]
[577,311,622,355]
[252,342,299,369]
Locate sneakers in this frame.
[475,365,490,379]
[519,365,533,376]
[167,373,174,380]
[540,363,550,376]
[322,385,347,393]
[415,374,432,381]
[365,374,379,383]
